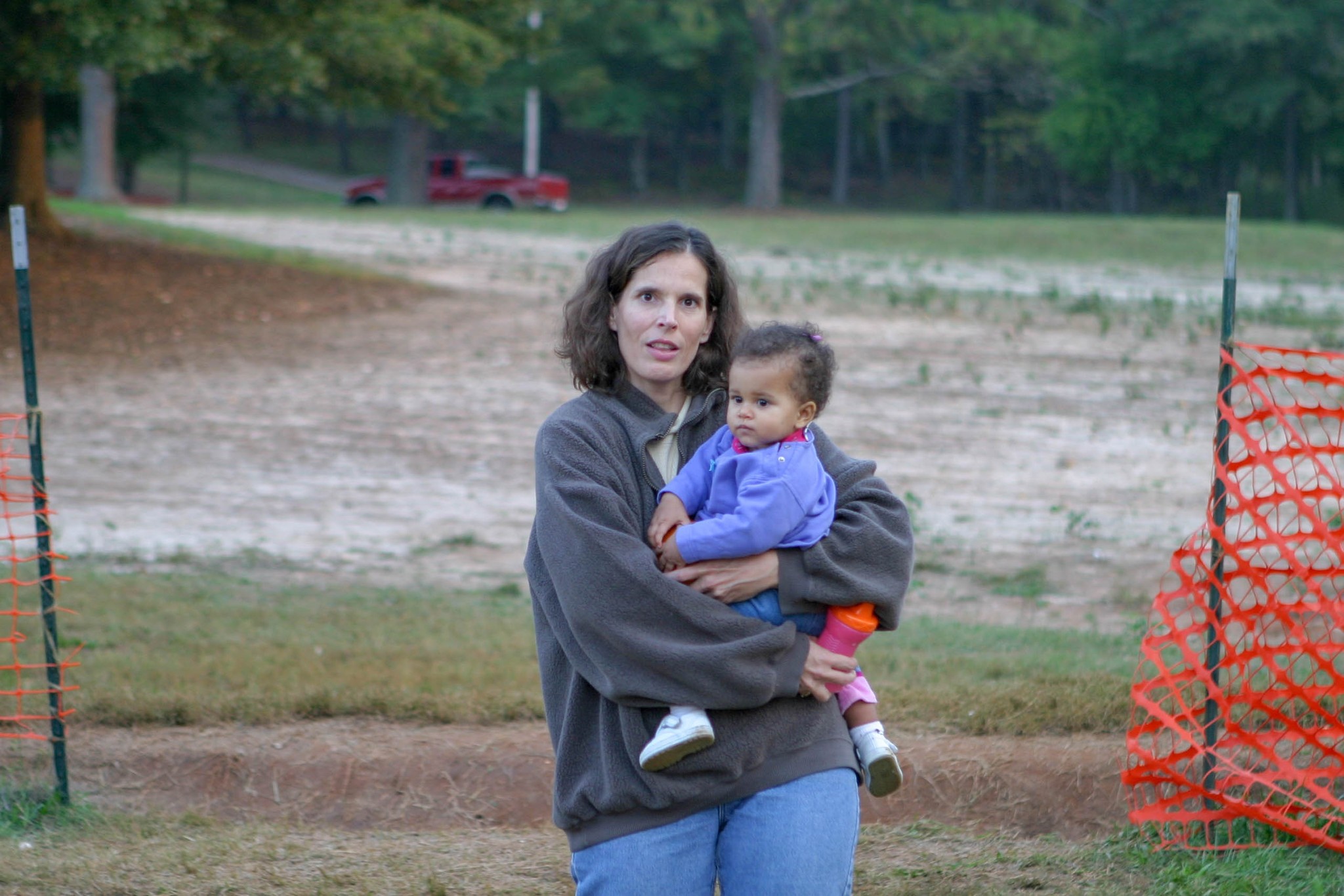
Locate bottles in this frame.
[814,601,879,694]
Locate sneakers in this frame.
[640,713,715,772]
[856,732,903,798]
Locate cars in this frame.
[344,149,571,212]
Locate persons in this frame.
[522,219,913,896]
[638,324,904,798]
